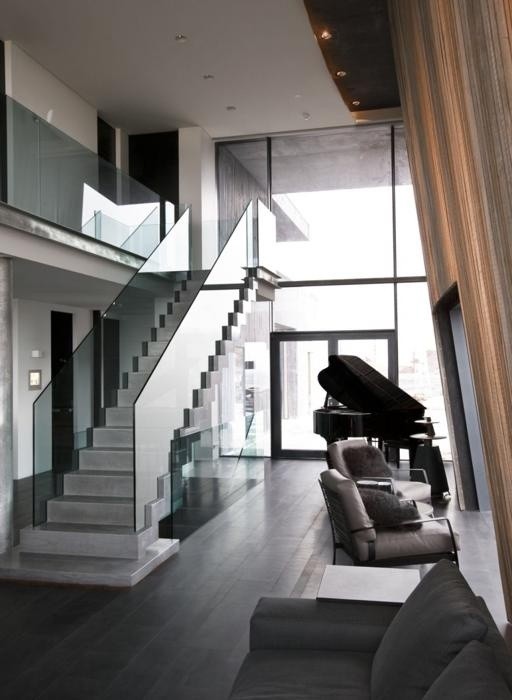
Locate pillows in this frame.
[368,557,509,699]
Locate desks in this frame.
[316,563,421,603]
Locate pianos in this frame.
[312,354,427,470]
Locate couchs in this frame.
[230,555,512,699]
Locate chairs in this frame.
[320,438,460,569]
